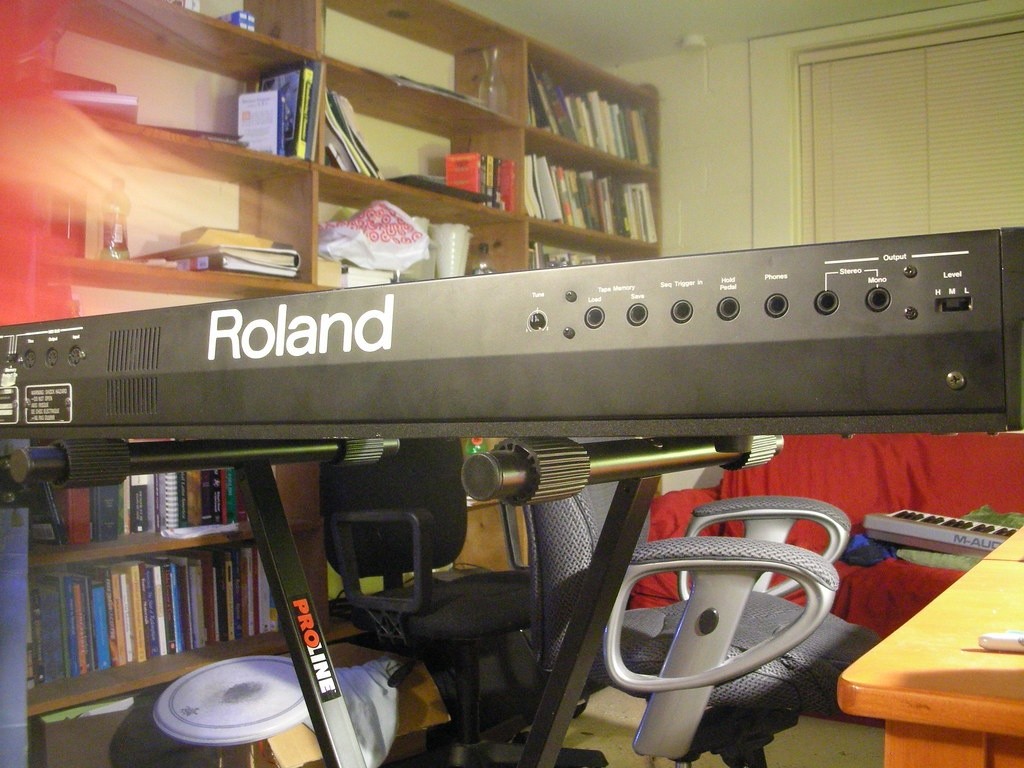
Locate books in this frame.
[135,227,301,278]
[27,467,280,689]
[237,58,385,180]
[446,56,657,270]
[23,67,139,124]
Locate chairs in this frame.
[316,436,607,768]
[524,435,883,768]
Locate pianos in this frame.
[863,509,1020,556]
[0,227,1024,768]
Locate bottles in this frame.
[470,242,498,276]
[97,176,130,261]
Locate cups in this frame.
[429,223,474,278]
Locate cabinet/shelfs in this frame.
[0,0,664,768]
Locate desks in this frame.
[836,523,1024,768]
